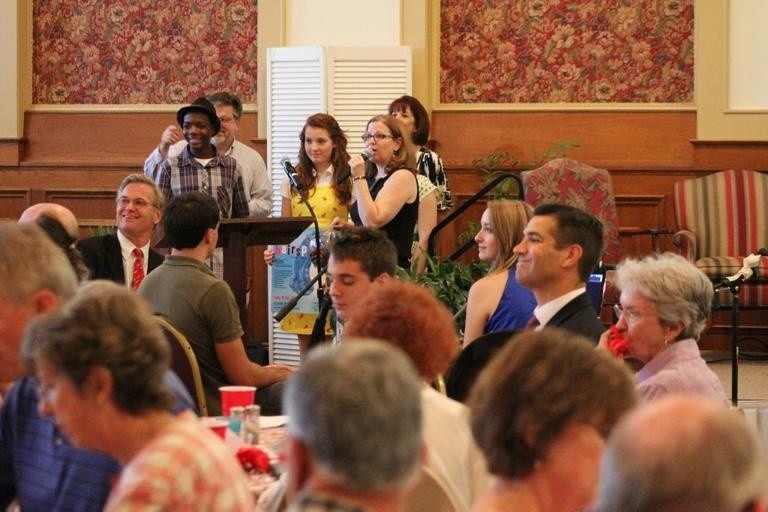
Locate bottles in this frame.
[228,404,260,445]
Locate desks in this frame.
[210,422,292,494]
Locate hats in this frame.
[175,97,220,138]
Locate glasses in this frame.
[611,304,660,322]
[117,197,154,210]
[361,132,396,143]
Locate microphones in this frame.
[281,156,303,189]
[335,149,371,184]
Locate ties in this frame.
[525,314,540,330]
[130,247,145,292]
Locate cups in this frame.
[219,385,257,417]
[202,419,229,440]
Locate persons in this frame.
[346,114,418,280]
[263,113,358,363]
[388,95,454,249]
[155,96,250,219]
[143,91,274,218]
[411,172,438,277]
[0,174,768,512]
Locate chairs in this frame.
[407,457,458,511]
[516,154,671,328]
[671,169,767,363]
